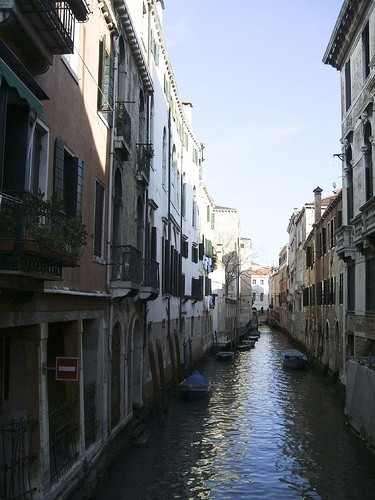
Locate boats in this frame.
[179,369,210,398]
[217,349,236,360]
[282,348,308,369]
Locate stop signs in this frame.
[54,356,79,382]
